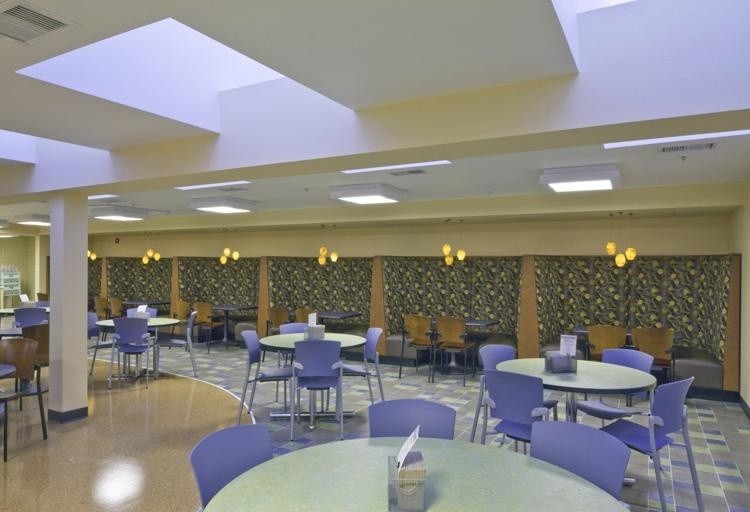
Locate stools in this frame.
[386,332,432,366]
[481,331,518,348]
[541,340,584,360]
[674,345,722,398]
[345,328,370,360]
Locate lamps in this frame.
[440,217,467,267]
[87,235,97,260]
[317,223,338,266]
[606,211,637,268]
[219,226,239,266]
[141,231,161,265]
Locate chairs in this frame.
[1,298,52,463]
[188,421,277,511]
[398,314,451,381]
[568,347,656,428]
[87,291,361,354]
[470,344,559,446]
[126,306,160,371]
[155,310,198,378]
[288,339,343,442]
[601,375,705,512]
[239,327,297,414]
[107,317,157,389]
[431,316,478,387]
[626,322,674,383]
[481,368,551,453]
[84,311,119,381]
[336,326,385,410]
[531,420,631,502]
[585,324,629,363]
[368,398,457,441]
[188,421,275,511]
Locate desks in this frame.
[495,357,656,427]
[97,318,181,379]
[432,319,495,354]
